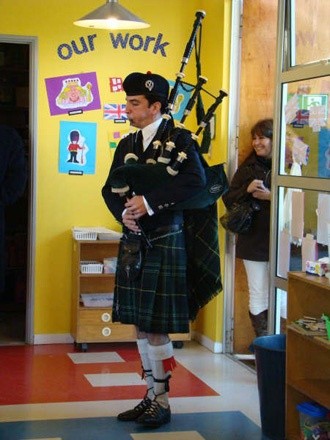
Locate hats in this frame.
[122,72,169,96]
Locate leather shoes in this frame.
[136,401,171,427]
[118,395,152,422]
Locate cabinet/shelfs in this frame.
[285,271,330,440]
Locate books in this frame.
[292,320,327,336]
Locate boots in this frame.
[248,309,268,338]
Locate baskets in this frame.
[72,229,98,240]
[79,260,104,273]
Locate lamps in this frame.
[73,0,150,30]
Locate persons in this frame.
[101,72,227,429]
[222,119,273,354]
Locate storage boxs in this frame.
[72,229,97,240]
[295,402,330,440]
[70,228,192,352]
[80,261,103,275]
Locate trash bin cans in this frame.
[252,334,287,440]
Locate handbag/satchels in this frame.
[220,164,261,235]
